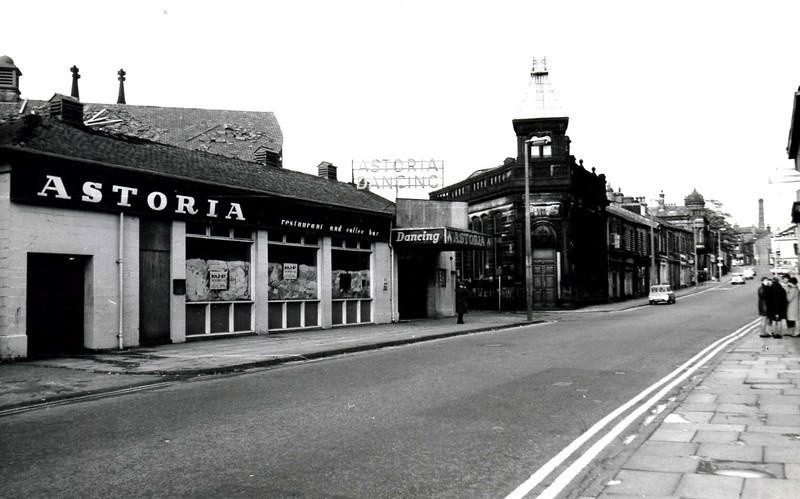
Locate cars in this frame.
[647,281,676,307]
[729,272,747,284]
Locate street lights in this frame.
[523,138,549,322]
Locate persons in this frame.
[758,273,800,339]
[455,281,468,324]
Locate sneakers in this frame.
[760,332,800,338]
